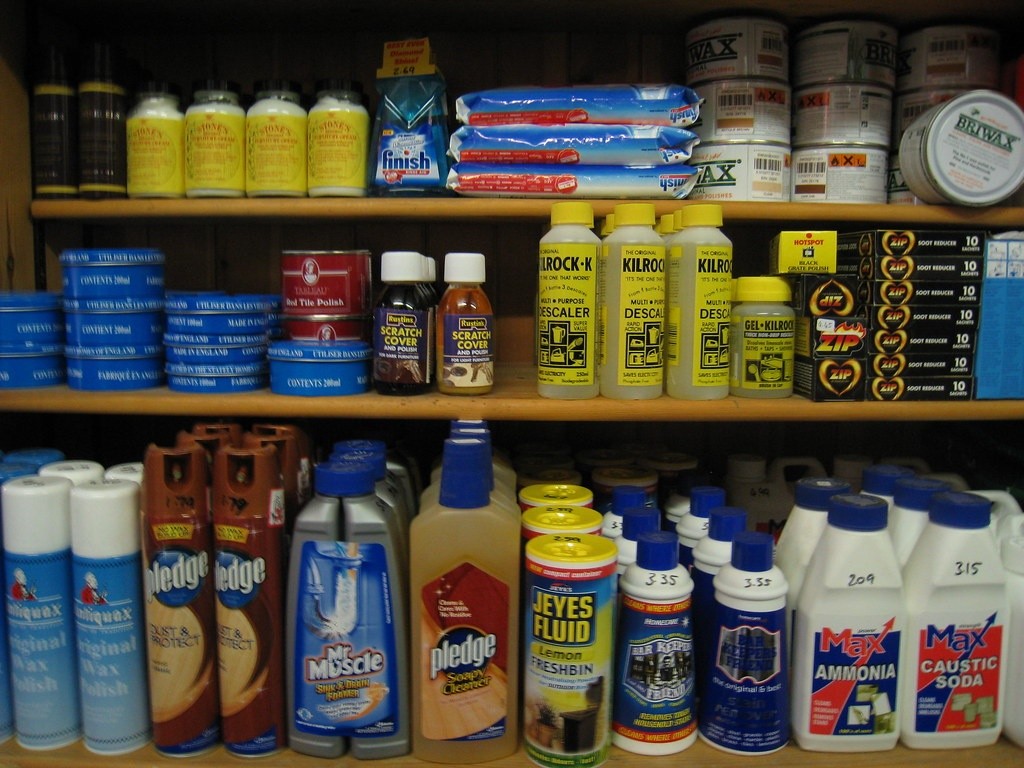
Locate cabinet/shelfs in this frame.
[0,0,1024,768]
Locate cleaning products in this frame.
[1,416,1023,768]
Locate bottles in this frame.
[31,36,368,197]
[284,420,517,764]
[0,447,154,756]
[536,202,799,399]
[368,251,499,395]
[609,451,1024,760]
